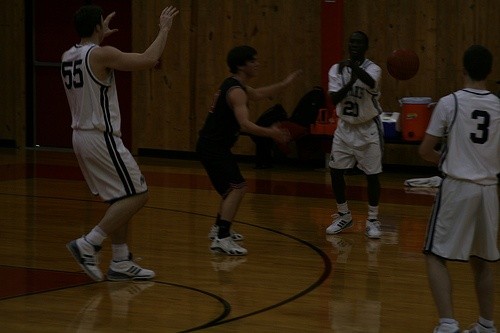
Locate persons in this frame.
[195,44,304,255]
[419,44,500,333]
[326,31,382,240]
[60,3,180,283]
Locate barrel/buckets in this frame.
[398,97,436,141]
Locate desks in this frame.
[302,133,441,169]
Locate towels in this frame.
[404,176,443,188]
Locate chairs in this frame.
[253,89,325,168]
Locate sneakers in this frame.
[423,322,460,333]
[66,234,104,283]
[107,259,155,281]
[208,226,244,241]
[462,323,496,333]
[364,219,382,239]
[326,212,353,234]
[210,236,248,255]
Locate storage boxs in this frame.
[379,111,400,138]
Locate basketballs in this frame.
[386,47,419,80]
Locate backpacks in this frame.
[291,86,324,127]
[249,105,287,142]
